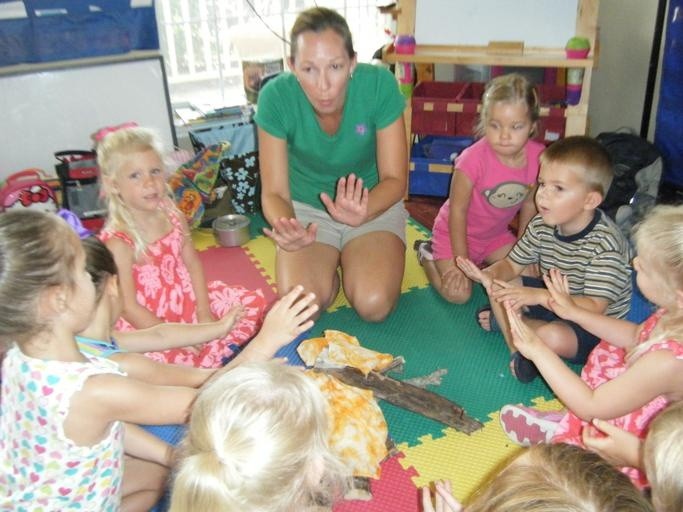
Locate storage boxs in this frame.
[407,82,565,199]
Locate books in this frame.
[187,106,253,125]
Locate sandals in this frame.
[413,240,433,265]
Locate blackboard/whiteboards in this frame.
[397,0,599,58]
[0,53,178,183]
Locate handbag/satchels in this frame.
[55,150,108,218]
[593,126,665,233]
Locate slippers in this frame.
[475,305,502,332]
[509,350,539,384]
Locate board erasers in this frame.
[488,41,523,58]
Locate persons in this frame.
[165,359,356,512]
[411,74,547,302]
[69,235,243,386]
[642,400,683,512]
[252,7,407,326]
[500,204,683,492]
[421,442,654,512]
[95,126,263,369]
[475,134,631,383]
[0,210,319,512]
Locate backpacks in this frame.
[1,170,59,215]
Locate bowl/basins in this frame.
[211,213,251,248]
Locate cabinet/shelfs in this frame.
[383,44,593,201]
